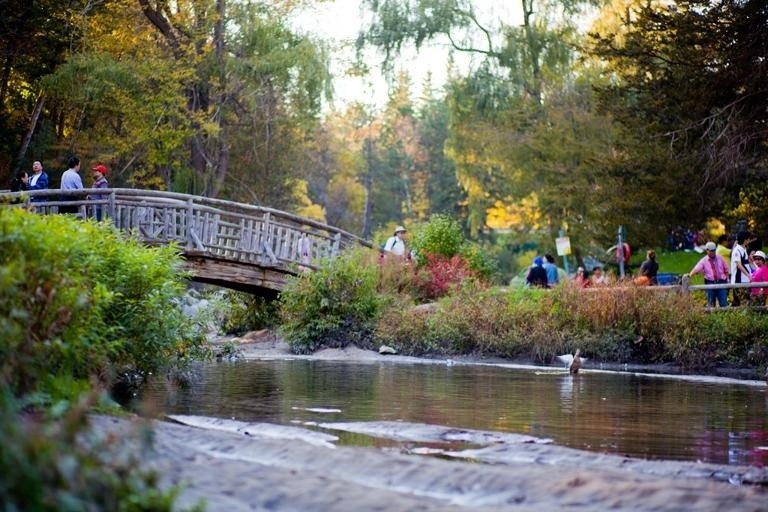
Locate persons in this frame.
[22,159,48,213]
[88,165,109,222]
[525,220,768,307]
[384,226,407,259]
[57,156,84,215]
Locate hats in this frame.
[395,226,405,232]
[754,251,765,259]
[93,166,106,173]
[706,242,716,250]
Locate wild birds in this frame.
[555,348,589,373]
